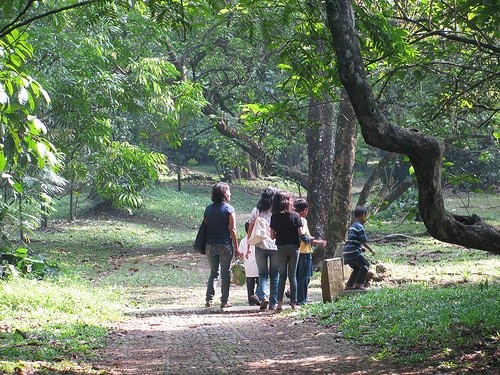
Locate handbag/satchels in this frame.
[193,221,208,255]
[247,207,267,245]
[232,264,245,286]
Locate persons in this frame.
[203,182,241,308]
[269,191,315,312]
[245,187,281,308]
[342,206,376,290]
[293,198,327,306]
[238,219,263,306]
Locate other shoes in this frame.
[353,285,365,290]
[251,294,263,304]
[205,301,213,306]
[260,299,268,309]
[221,302,232,307]
[345,285,355,290]
